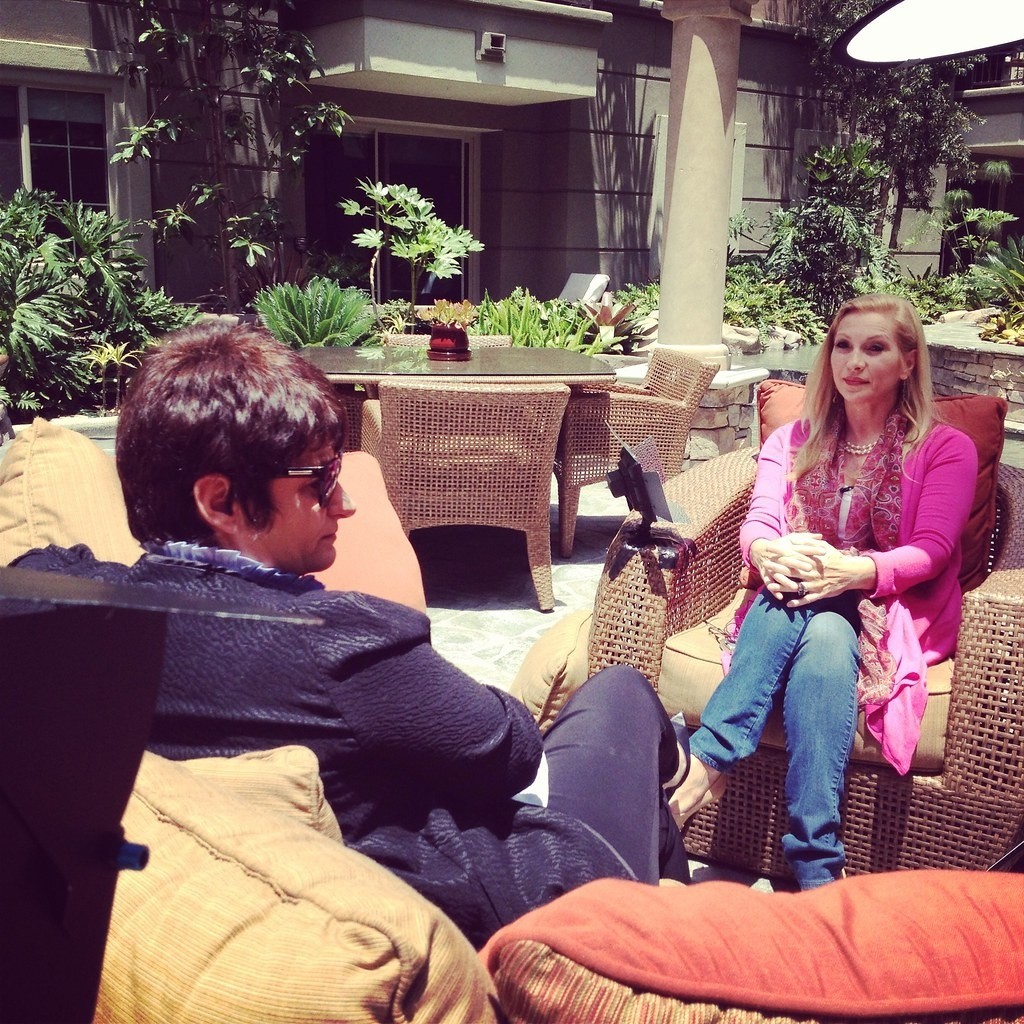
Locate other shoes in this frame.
[668,768,728,829]
[661,711,692,801]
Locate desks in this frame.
[294,344,619,553]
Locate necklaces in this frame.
[843,440,878,455]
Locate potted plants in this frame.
[418,299,480,350]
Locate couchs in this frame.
[0,415,1024,1024]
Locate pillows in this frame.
[738,379,1008,594]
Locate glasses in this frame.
[273,450,343,508]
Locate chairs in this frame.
[360,378,573,612]
[558,273,611,302]
[553,345,721,560]
[592,446,1024,879]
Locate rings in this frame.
[797,581,806,597]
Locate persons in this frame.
[6,326,693,954]
[666,296,978,893]
